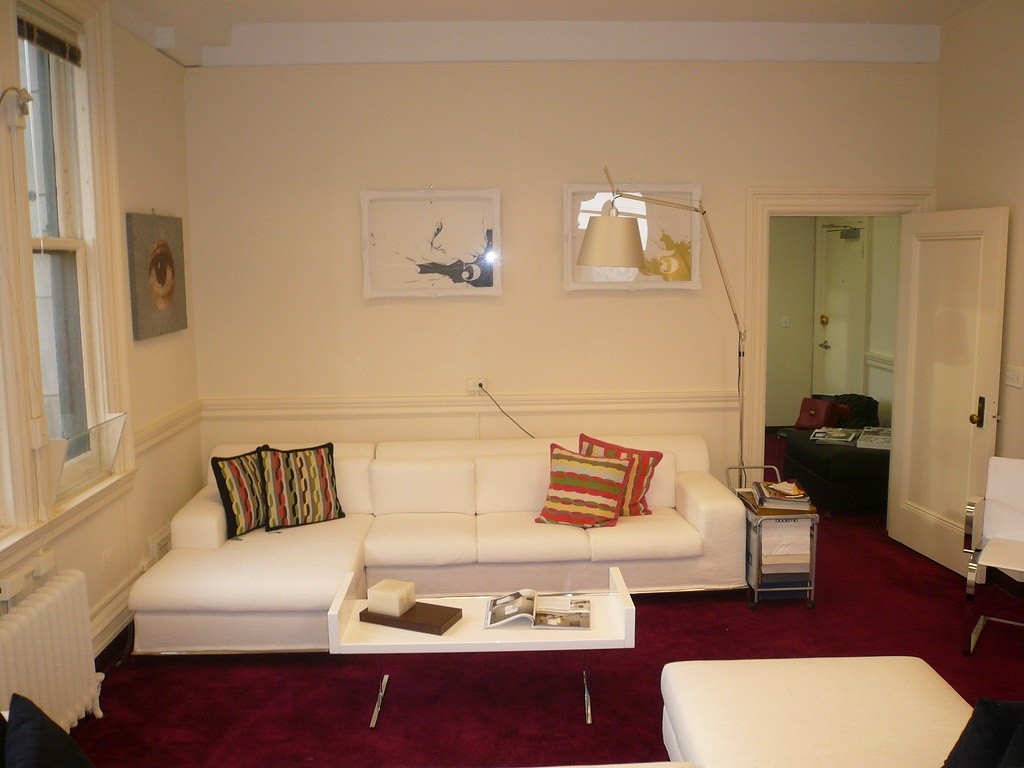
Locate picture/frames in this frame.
[360,189,504,298]
[562,182,702,292]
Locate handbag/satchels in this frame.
[794,396,849,429]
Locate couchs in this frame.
[783,429,890,519]
[128,435,749,656]
[660,656,972,768]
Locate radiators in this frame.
[0,570,105,735]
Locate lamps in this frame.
[577,189,747,490]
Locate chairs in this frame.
[776,395,832,467]
[963,457,1024,602]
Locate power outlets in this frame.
[467,378,486,391]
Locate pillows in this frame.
[212,445,270,539]
[257,443,344,531]
[578,434,663,516]
[535,443,635,529]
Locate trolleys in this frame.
[725,465,821,610]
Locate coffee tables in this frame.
[329,567,636,725]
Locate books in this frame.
[482,589,591,630]
[752,481,810,511]
